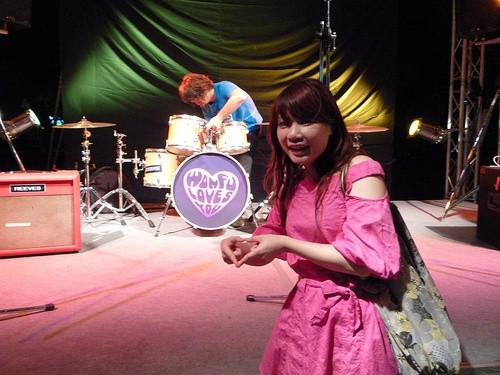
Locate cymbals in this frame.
[52,121,117,129]
[345,125,389,133]
[254,121,271,127]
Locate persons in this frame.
[178,73,264,178]
[219,79,401,375]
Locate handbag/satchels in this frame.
[340,162,461,375]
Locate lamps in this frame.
[1,109,40,139]
[408,120,447,144]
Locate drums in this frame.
[165,114,209,156]
[170,152,253,230]
[89,166,140,214]
[215,120,251,156]
[142,148,185,189]
